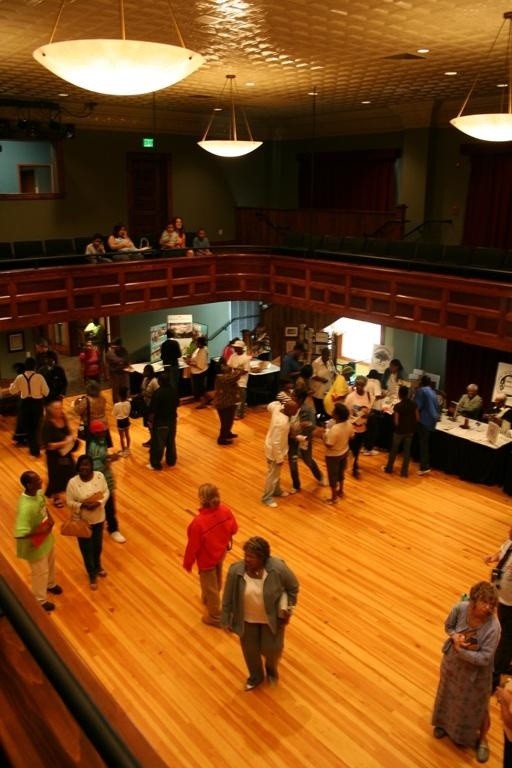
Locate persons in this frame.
[183,484,238,627]
[221,536,299,689]
[14,470,63,611]
[431,580,503,762]
[495,677,512,768]
[66,455,113,589]
[83,218,215,263]
[484,527,512,695]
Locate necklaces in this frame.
[255,572,260,576]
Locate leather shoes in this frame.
[218,441,232,444]
[228,434,238,438]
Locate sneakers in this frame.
[433,727,446,739]
[246,679,257,689]
[318,472,346,507]
[47,585,63,595]
[194,398,214,410]
[281,492,289,497]
[269,674,278,686]
[351,463,432,479]
[98,570,108,577]
[110,531,127,543]
[42,601,55,611]
[359,445,380,455]
[200,613,223,628]
[114,437,178,471]
[290,488,299,494]
[268,502,278,508]
[90,583,98,591]
[476,736,490,764]
[54,497,65,508]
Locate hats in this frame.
[340,365,356,376]
[231,340,245,348]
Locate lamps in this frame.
[196,73,264,159]
[31,0,207,98]
[448,10,512,142]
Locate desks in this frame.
[371,392,512,496]
[128,356,209,401]
[209,354,281,408]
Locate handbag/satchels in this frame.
[137,236,153,255]
[59,503,94,539]
[76,422,91,443]
[441,605,461,656]
[489,567,503,589]
[277,590,289,620]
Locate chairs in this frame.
[0,235,115,269]
